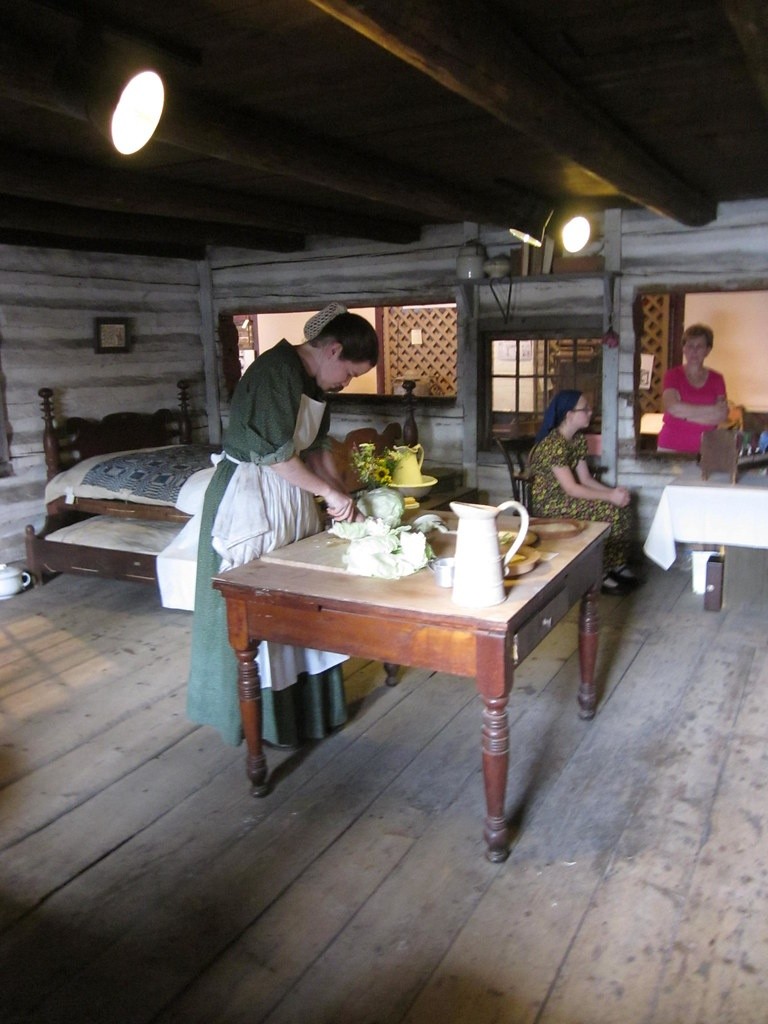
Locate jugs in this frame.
[393,444,425,487]
[450,501,530,610]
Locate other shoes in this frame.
[606,565,638,587]
[600,574,630,596]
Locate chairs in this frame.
[494,438,607,515]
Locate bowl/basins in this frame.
[482,258,510,278]
[389,474,438,498]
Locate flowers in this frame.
[350,442,402,491]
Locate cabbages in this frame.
[326,516,435,579]
[356,487,404,518]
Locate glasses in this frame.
[569,403,590,413]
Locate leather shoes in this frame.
[263,739,304,752]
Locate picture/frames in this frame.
[93,316,130,354]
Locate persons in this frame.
[185,302,379,746]
[657,324,728,452]
[528,389,638,596]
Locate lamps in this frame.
[509,202,592,252]
[87,43,166,156]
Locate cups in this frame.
[427,556,454,588]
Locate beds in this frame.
[24,381,416,586]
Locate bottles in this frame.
[455,244,487,280]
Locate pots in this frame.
[393,370,431,397]
[0,564,31,600]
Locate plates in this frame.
[518,519,586,539]
[499,546,540,576]
[496,527,538,547]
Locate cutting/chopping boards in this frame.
[259,529,375,577]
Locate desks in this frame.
[644,471,768,611]
[207,509,613,866]
